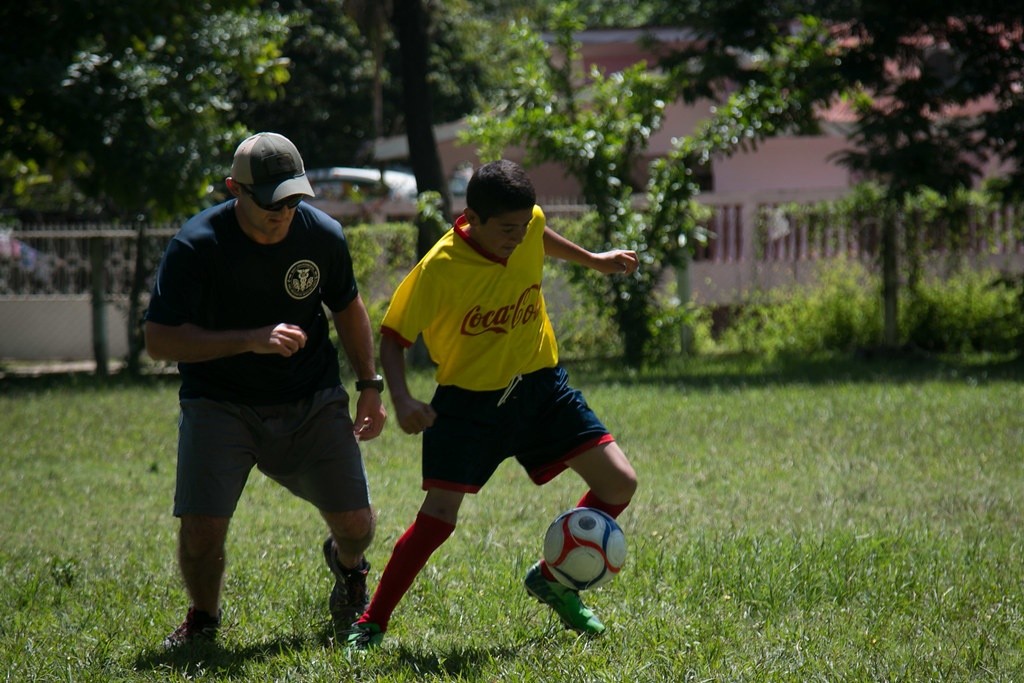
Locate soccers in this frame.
[543,506,628,591]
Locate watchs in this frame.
[355,375,384,393]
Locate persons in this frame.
[345,161,639,665]
[139,132,386,666]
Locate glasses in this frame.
[239,183,303,211]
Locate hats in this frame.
[231,132,315,204]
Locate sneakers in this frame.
[324,536,372,621]
[347,623,384,653]
[162,606,221,651]
[524,560,605,640]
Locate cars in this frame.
[302,166,470,227]
